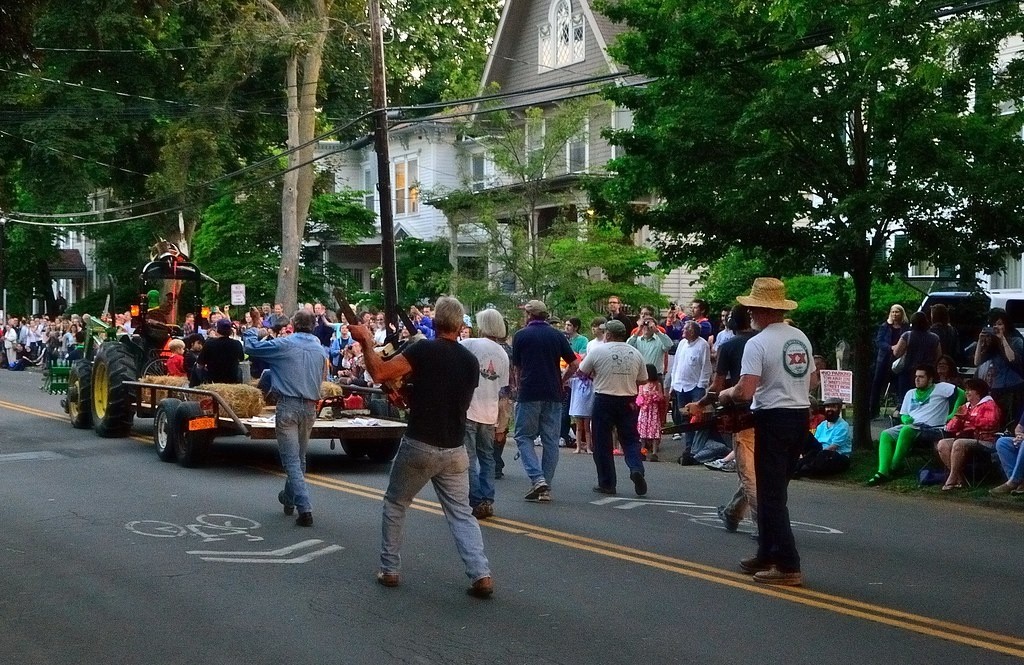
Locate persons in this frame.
[685,302,760,542]
[347,297,493,597]
[576,319,648,496]
[0,284,1024,494]
[243,306,325,526]
[512,300,579,502]
[459,308,509,519]
[716,278,817,585]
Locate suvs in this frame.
[910,288,1024,348]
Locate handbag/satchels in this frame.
[891,349,908,373]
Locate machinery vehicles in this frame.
[67,261,203,439]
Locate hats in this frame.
[217,318,232,335]
[736,277,798,311]
[823,397,843,407]
[599,320,627,337]
[146,290,160,308]
[549,316,561,323]
[518,299,549,317]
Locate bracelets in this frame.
[655,329,659,333]
[1003,345,1010,347]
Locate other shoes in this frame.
[466,577,493,598]
[718,498,739,531]
[720,460,738,472]
[1010,487,1024,494]
[630,470,648,495]
[377,571,400,587]
[890,469,905,478]
[524,479,549,500]
[471,502,493,518]
[749,533,760,540]
[738,554,770,573]
[753,565,805,585]
[278,489,295,515]
[538,490,552,501]
[704,459,725,470]
[295,512,314,525]
[593,485,617,494]
[495,472,503,479]
[988,483,1015,495]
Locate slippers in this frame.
[941,480,964,490]
[866,472,894,486]
